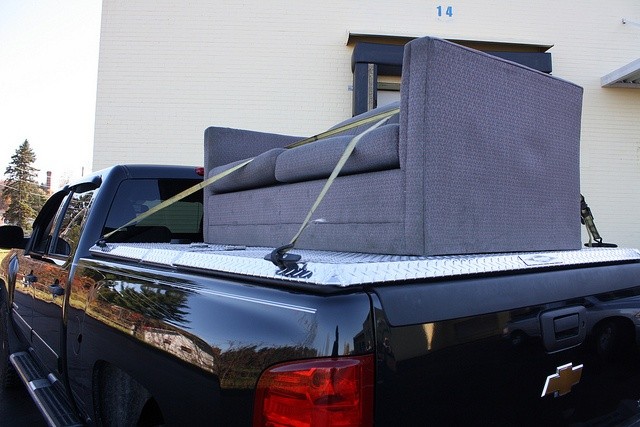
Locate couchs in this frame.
[202,35,584,257]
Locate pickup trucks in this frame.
[0,166,638,425]
